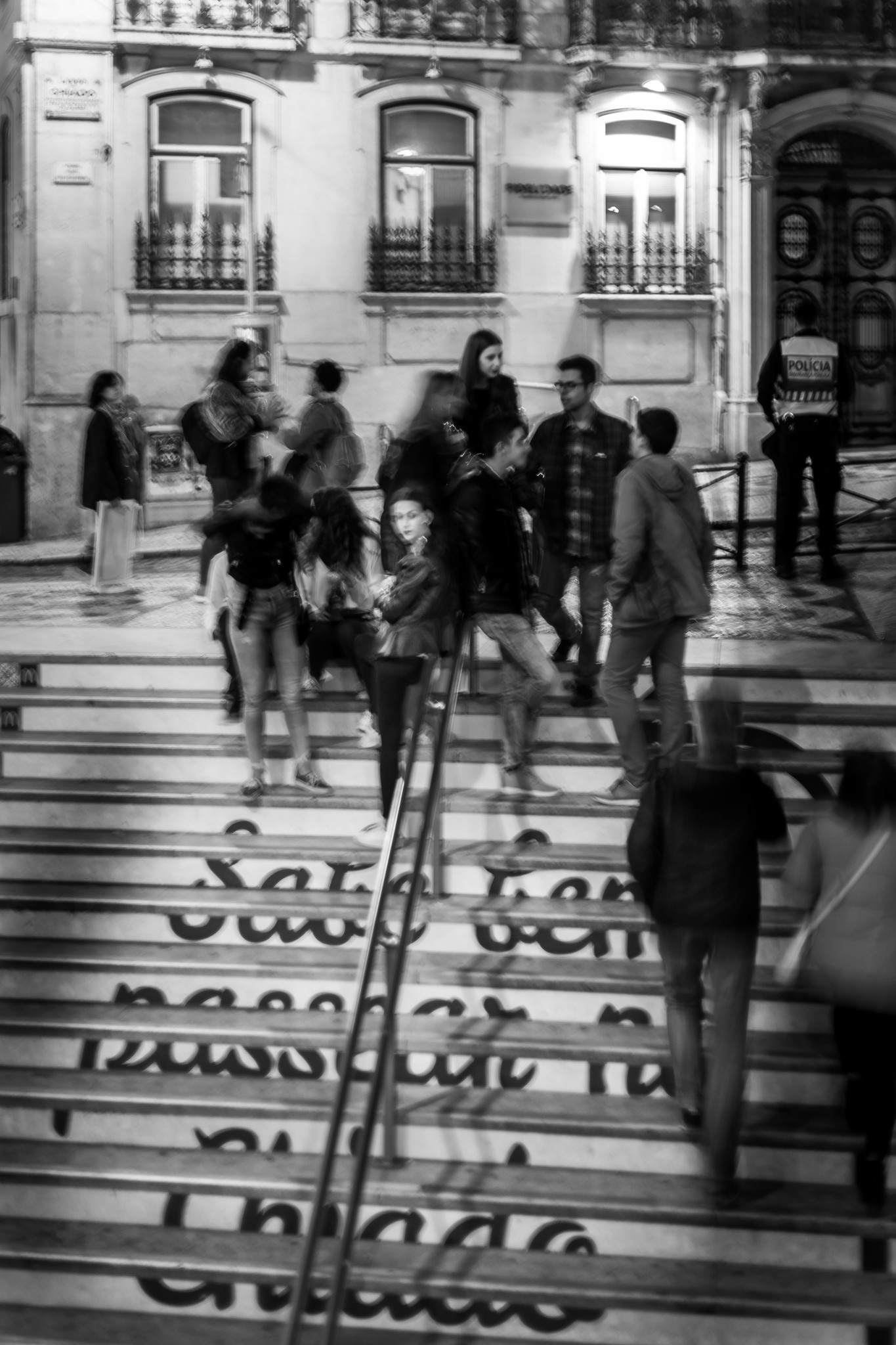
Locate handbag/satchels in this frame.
[773,916,813,986]
[181,402,229,464]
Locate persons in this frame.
[200,493,277,700]
[592,406,717,806]
[625,689,790,1210]
[79,371,148,595]
[354,488,444,850]
[787,736,896,1206]
[205,339,284,498]
[378,369,469,576]
[756,300,856,584]
[446,408,564,796]
[284,357,351,539]
[516,354,635,707]
[204,476,332,796]
[295,486,383,701]
[459,329,529,460]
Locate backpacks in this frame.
[315,400,365,487]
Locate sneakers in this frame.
[524,711,541,751]
[502,767,559,797]
[595,776,652,805]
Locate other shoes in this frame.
[570,681,595,707]
[704,1161,733,1189]
[552,619,581,661]
[772,559,796,577]
[678,1104,704,1132]
[296,771,333,797]
[243,775,266,797]
[355,688,371,700]
[303,677,321,698]
[821,564,848,581]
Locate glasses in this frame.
[553,380,590,392]
